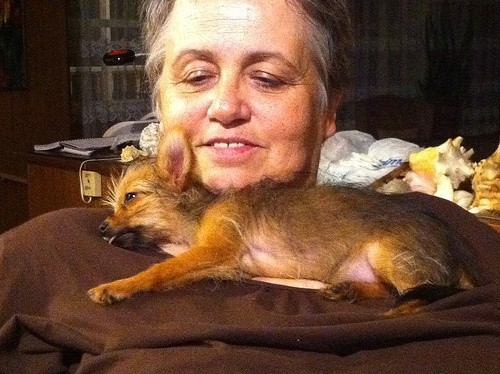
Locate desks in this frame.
[9,152,124,221]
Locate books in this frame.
[33,136,120,158]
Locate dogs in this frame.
[87,126,475,315]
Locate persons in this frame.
[137,0,336,192]
